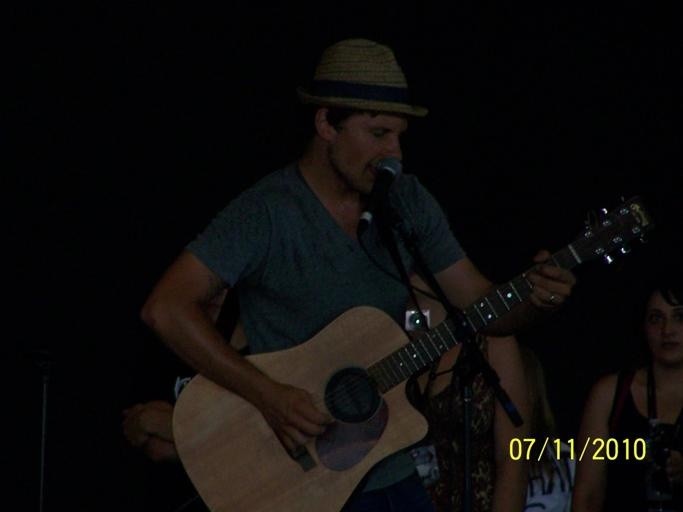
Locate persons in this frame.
[399,226,540,512]
[109,280,249,511]
[134,34,578,512]
[522,365,578,512]
[564,273,683,512]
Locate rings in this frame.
[546,294,556,304]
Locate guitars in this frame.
[171,196,653,512]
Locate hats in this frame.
[293,35,430,119]
